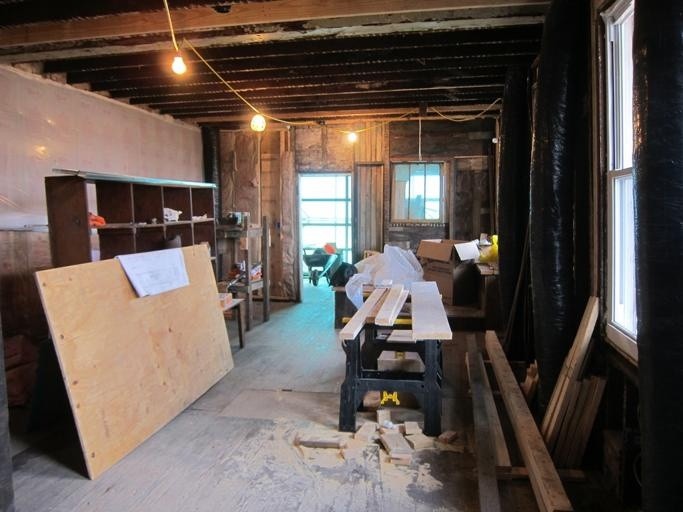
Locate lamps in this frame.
[171,49,187,76]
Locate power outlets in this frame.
[250,114,266,133]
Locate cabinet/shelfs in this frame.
[44,175,219,285]
[217,215,271,330]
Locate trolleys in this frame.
[303,246,342,286]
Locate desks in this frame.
[476,245,499,315]
[333,285,376,329]
[222,299,246,349]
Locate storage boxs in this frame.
[416,239,478,307]
